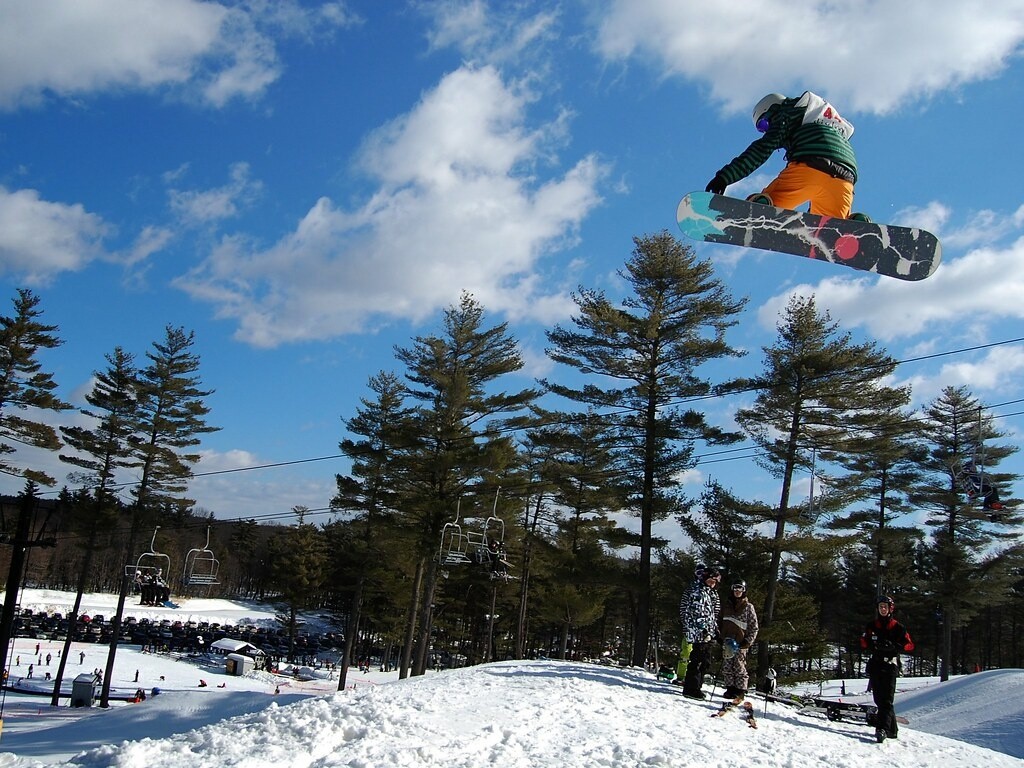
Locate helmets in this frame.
[876,594,894,615]
[695,563,707,573]
[752,89,788,132]
[732,578,746,591]
[703,567,720,585]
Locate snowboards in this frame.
[676,190,944,281]
[796,697,909,726]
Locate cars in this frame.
[13,602,394,657]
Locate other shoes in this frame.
[684,688,706,701]
[887,730,897,738]
[853,212,870,223]
[724,687,745,701]
[876,727,886,743]
[754,195,770,206]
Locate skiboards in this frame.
[710,697,758,730]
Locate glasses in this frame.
[695,569,705,576]
[758,120,770,133]
[735,583,746,593]
[716,575,723,584]
[876,597,891,604]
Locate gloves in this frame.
[739,641,748,650]
[705,174,727,195]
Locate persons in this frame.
[302,656,337,671]
[200,680,207,686]
[3,669,8,684]
[17,642,85,680]
[717,579,759,701]
[135,687,162,703]
[860,595,914,744]
[94,668,103,685]
[488,539,509,585]
[254,662,279,674]
[293,667,299,674]
[136,567,170,607]
[680,568,720,701]
[133,670,139,682]
[672,563,720,686]
[705,90,870,223]
[275,686,280,694]
[160,676,165,681]
[359,658,392,674]
